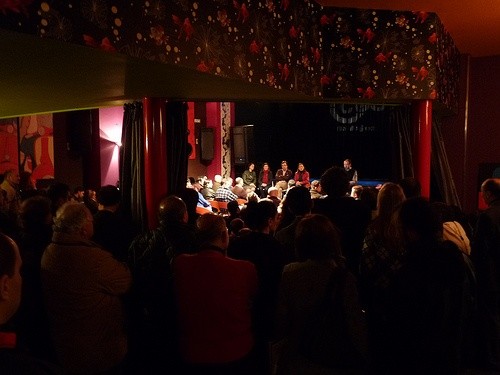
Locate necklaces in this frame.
[197,241,228,257]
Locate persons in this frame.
[0,167,483,364]
[293,163,311,190]
[242,163,256,194]
[38,201,133,375]
[274,214,369,375]
[274,161,293,191]
[172,214,260,375]
[342,159,358,196]
[384,200,466,375]
[0,231,66,375]
[258,162,273,199]
[473,176,500,370]
